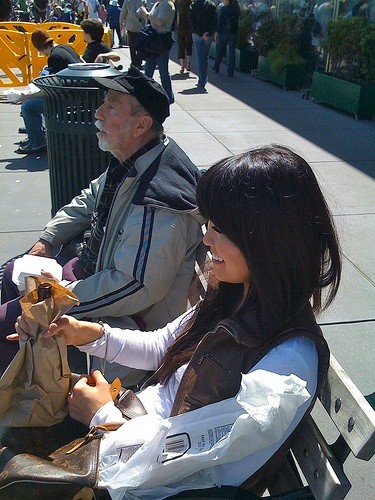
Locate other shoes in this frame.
[21,137,30,145]
[20,127,27,132]
[180,67,184,74]
[19,143,48,154]
[187,65,191,71]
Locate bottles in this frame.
[37,283,52,302]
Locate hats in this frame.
[32,28,55,51]
[109,0,121,6]
[91,64,171,122]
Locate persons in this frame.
[14,18,120,153]
[0,0,107,43]
[0,66,209,388]
[5,145,342,500]
[105,0,241,103]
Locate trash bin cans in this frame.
[33,62,125,217]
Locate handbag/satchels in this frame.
[0,374,148,499]
[135,25,157,50]
[163,31,176,53]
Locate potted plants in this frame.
[251,19,310,91]
[211,10,257,71]
[309,16,375,119]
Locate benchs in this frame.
[186,218,375,499]
